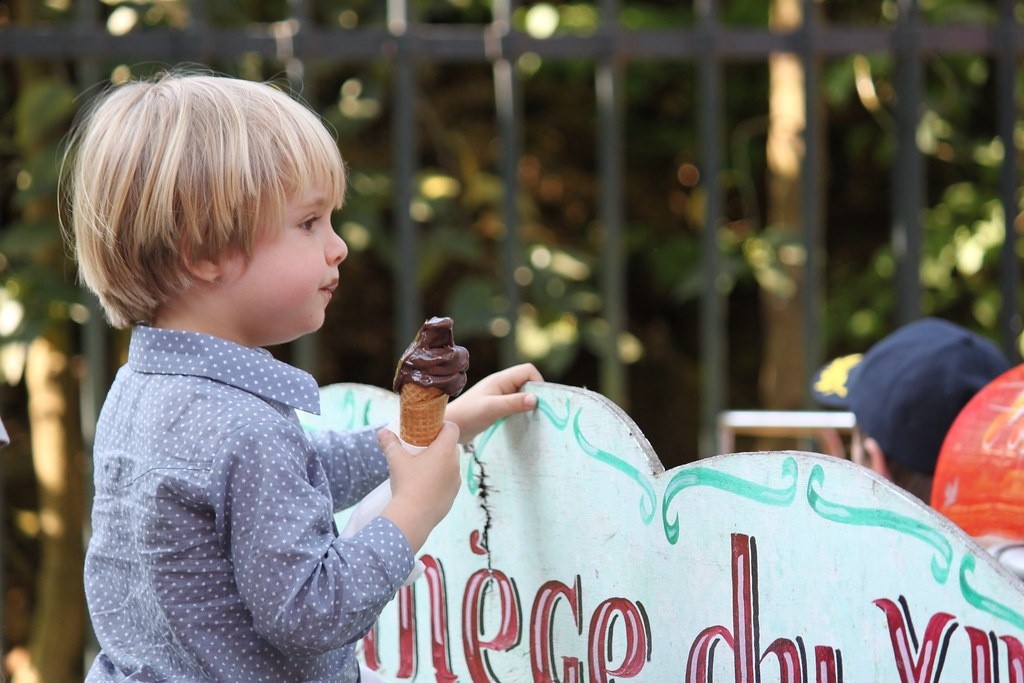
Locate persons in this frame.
[812,320,1009,505]
[73,75,544,683]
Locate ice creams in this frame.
[392,316,469,447]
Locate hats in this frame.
[812,319,1010,472]
